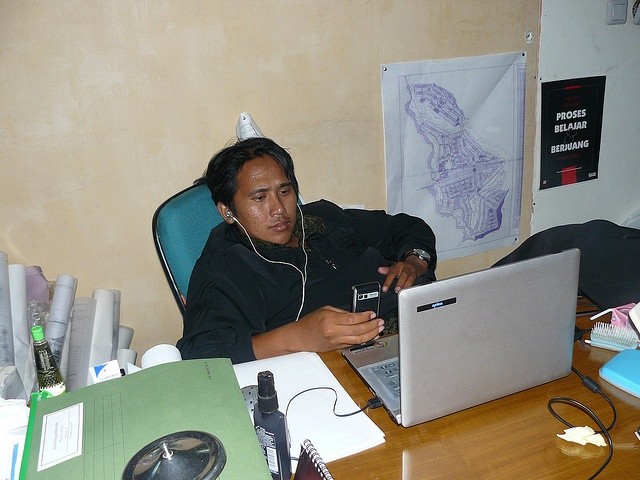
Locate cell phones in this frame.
[350,281,381,318]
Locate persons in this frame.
[175,136,438,364]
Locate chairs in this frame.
[151,179,303,318]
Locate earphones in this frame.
[226,211,231,217]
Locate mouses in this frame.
[240,385,258,427]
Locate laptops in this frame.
[339,247,581,428]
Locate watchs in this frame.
[404,248,432,264]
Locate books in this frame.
[292,440,335,479]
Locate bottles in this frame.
[253,370,291,480]
[31,325,66,398]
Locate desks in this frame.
[290,295,639,479]
